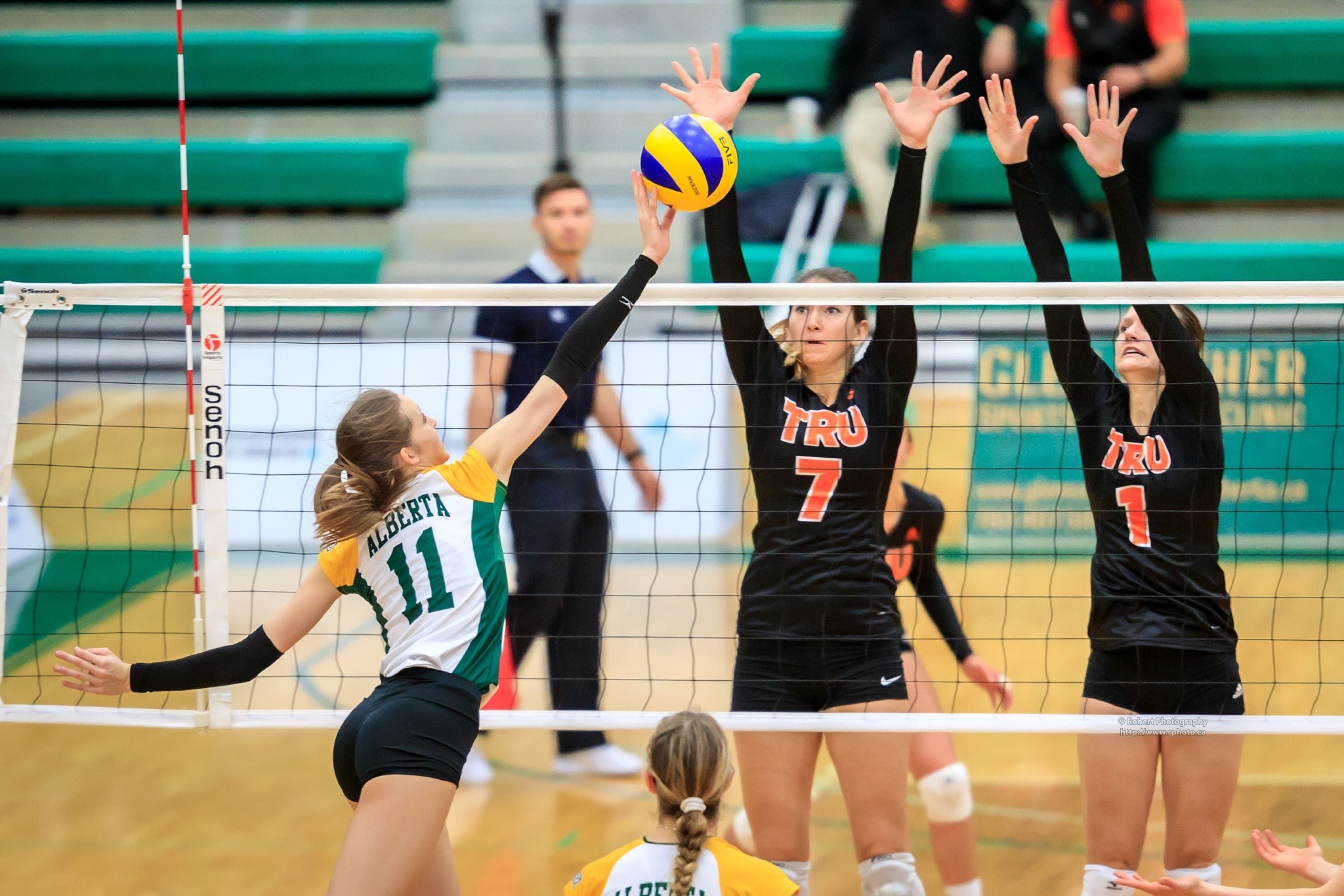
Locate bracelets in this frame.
[623,447,644,463]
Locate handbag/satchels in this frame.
[738,171,830,243]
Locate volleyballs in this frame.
[641,114,739,212]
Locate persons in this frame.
[725,420,1014,896]
[1113,829,1344,896]
[979,73,1247,896]
[54,170,679,896]
[564,708,802,896]
[458,173,671,783]
[660,43,970,896]
[815,1,1193,249]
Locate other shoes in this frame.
[459,750,497,782]
[553,744,643,777]
[1072,211,1113,242]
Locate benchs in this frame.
[0,0,1344,317]
